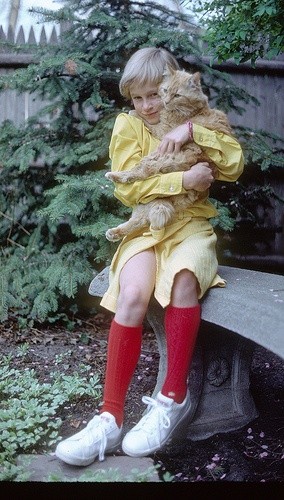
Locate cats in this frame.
[103,65,237,244]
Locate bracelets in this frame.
[189,121,193,142]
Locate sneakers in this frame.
[55,412,123,466]
[122,386,192,458]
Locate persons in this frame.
[55,49,244,467]
